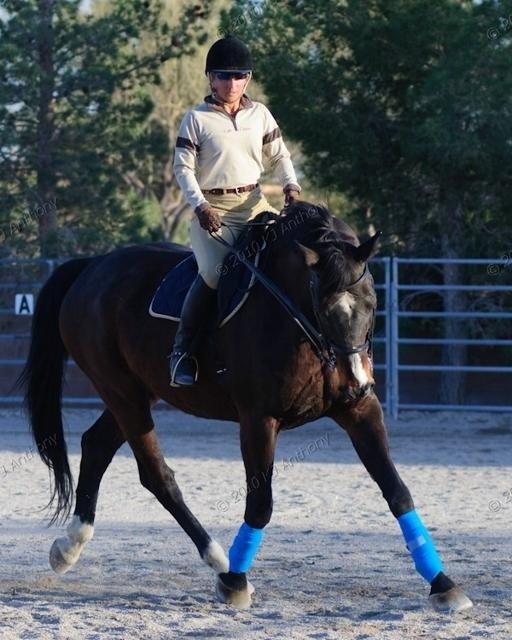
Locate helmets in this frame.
[205,38,253,76]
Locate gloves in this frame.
[283,183,299,204]
[193,203,223,229]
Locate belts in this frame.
[202,183,259,195]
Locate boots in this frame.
[170,272,216,386]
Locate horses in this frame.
[7,197,476,619]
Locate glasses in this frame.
[215,72,248,81]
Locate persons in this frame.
[170,38,302,386]
